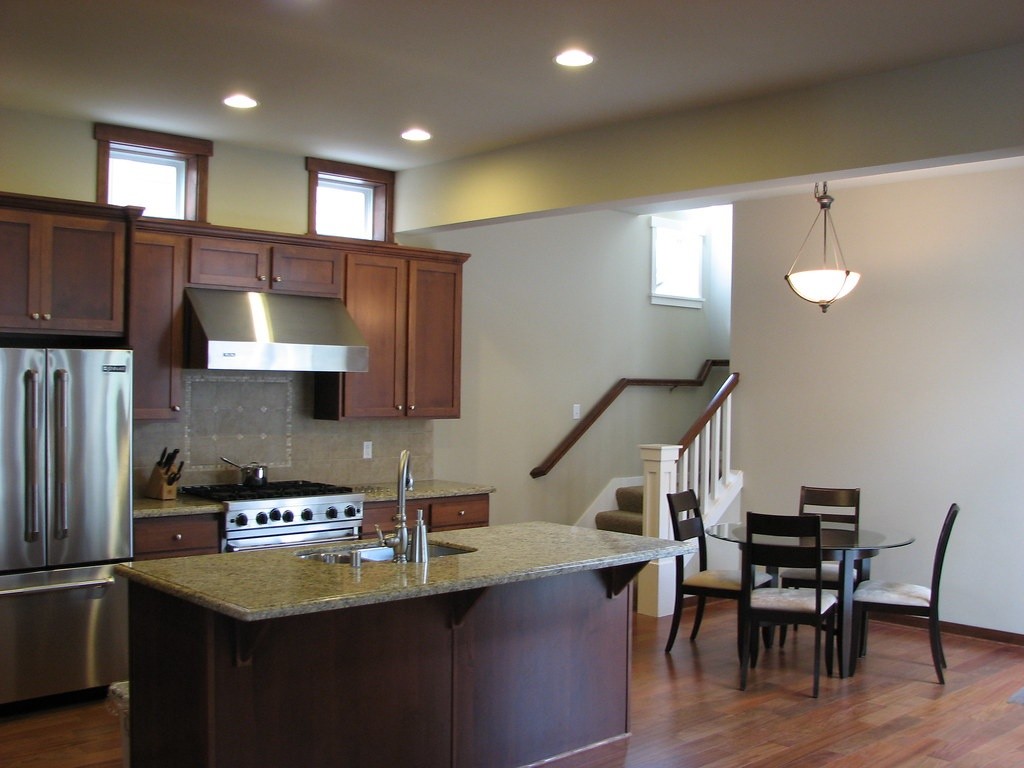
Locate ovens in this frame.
[222,526,362,552]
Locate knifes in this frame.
[158,447,184,474]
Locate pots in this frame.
[222,458,268,486]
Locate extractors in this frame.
[186,288,369,372]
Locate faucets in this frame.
[373,448,416,564]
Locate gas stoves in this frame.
[183,480,365,531]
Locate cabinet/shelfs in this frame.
[184,220,345,302]
[132,511,221,561]
[124,215,184,420]
[358,493,490,538]
[0,191,145,341]
[309,236,471,422]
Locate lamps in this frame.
[784,180,862,315]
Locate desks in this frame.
[703,522,918,678]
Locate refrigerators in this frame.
[0,339,130,703]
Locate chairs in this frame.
[738,510,838,699]
[662,488,773,668]
[848,503,961,685]
[776,485,861,648]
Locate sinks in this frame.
[295,551,371,565]
[347,539,480,564]
